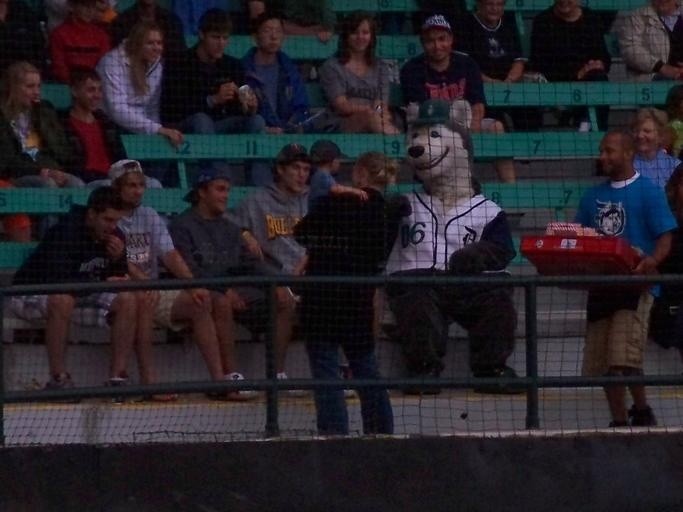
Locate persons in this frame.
[0,0,682,435]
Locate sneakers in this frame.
[276,376,312,399]
[44,377,80,404]
[406,359,443,396]
[607,400,658,428]
[465,365,526,396]
[104,378,144,404]
[148,382,178,401]
[223,371,256,400]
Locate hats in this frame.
[413,98,452,125]
[310,138,351,165]
[419,14,453,35]
[109,158,143,182]
[274,143,312,166]
[183,168,233,203]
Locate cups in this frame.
[237,85,255,96]
